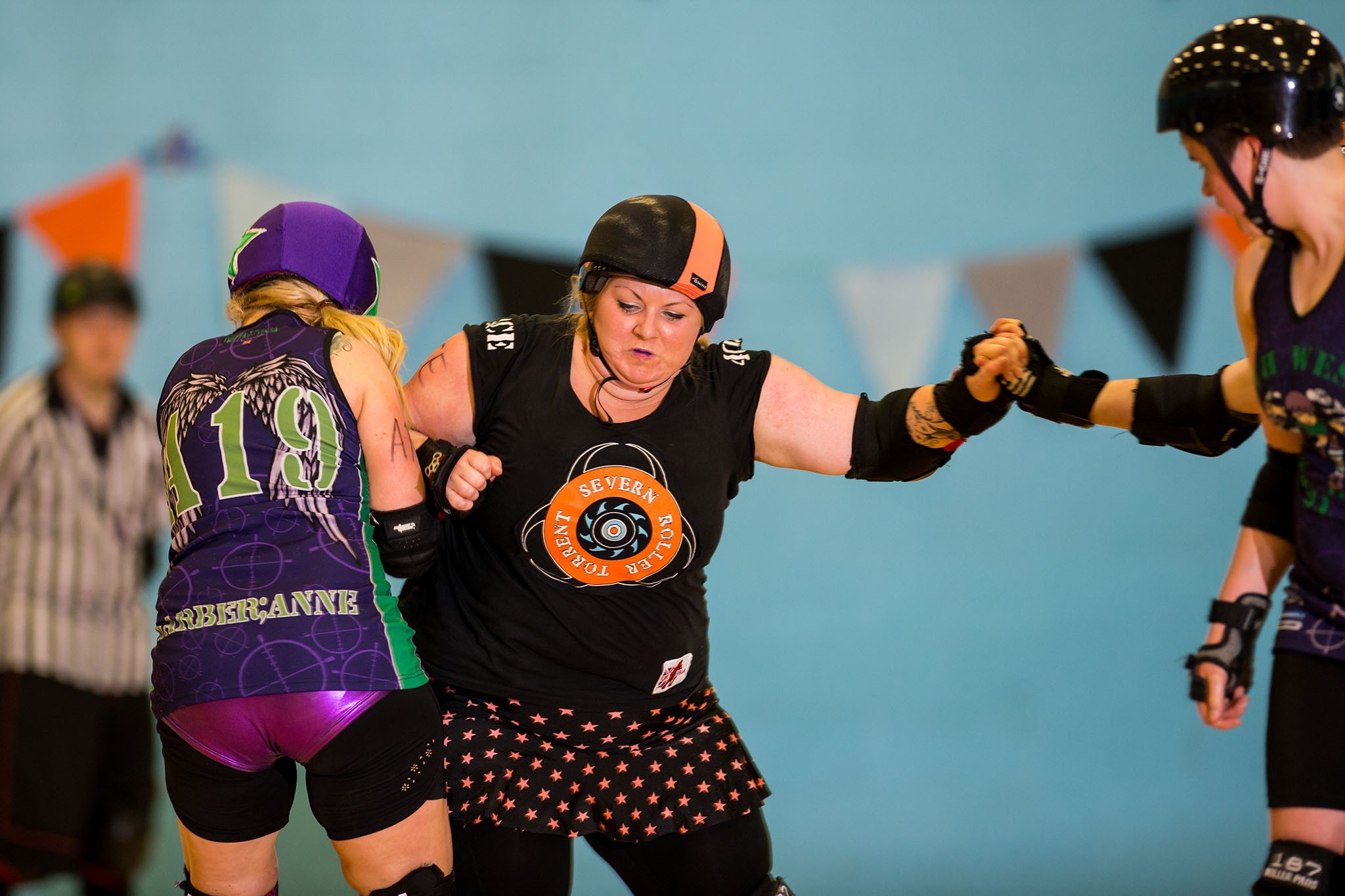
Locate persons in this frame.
[399,193,1028,896]
[148,203,454,896]
[0,255,169,896]
[973,13,1345,896]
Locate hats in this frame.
[53,266,135,322]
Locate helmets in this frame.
[578,194,732,334]
[226,202,379,319]
[1158,16,1345,140]
